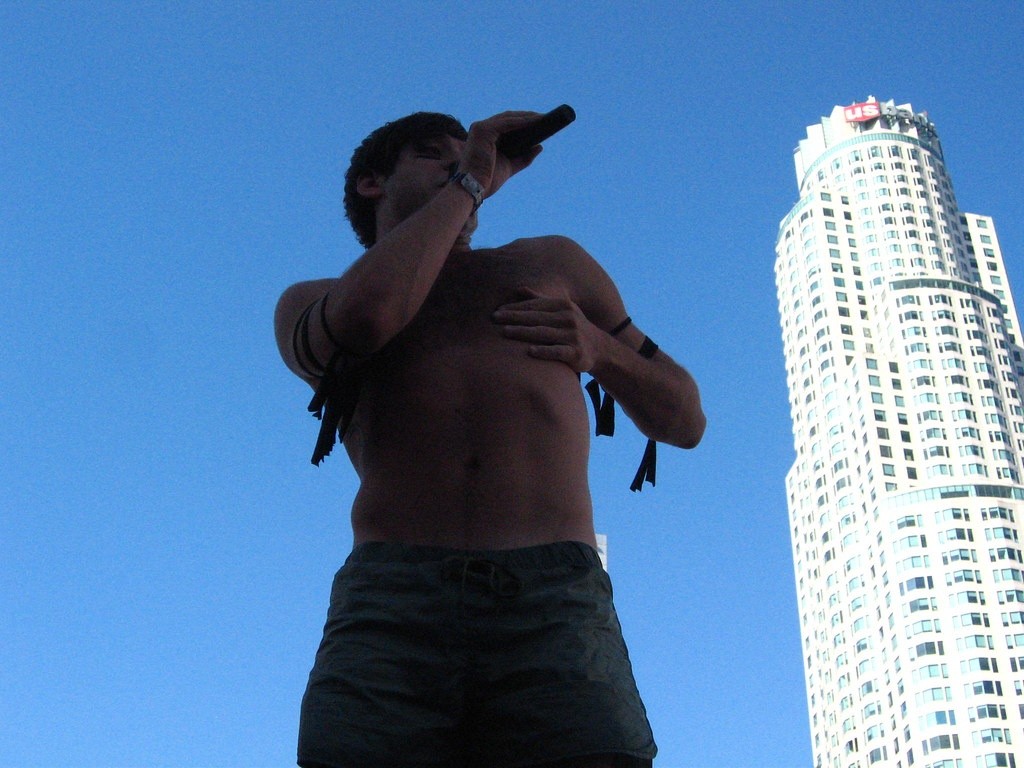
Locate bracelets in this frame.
[452,171,483,216]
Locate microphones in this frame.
[494,104,576,161]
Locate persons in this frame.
[274,112,707,768]
